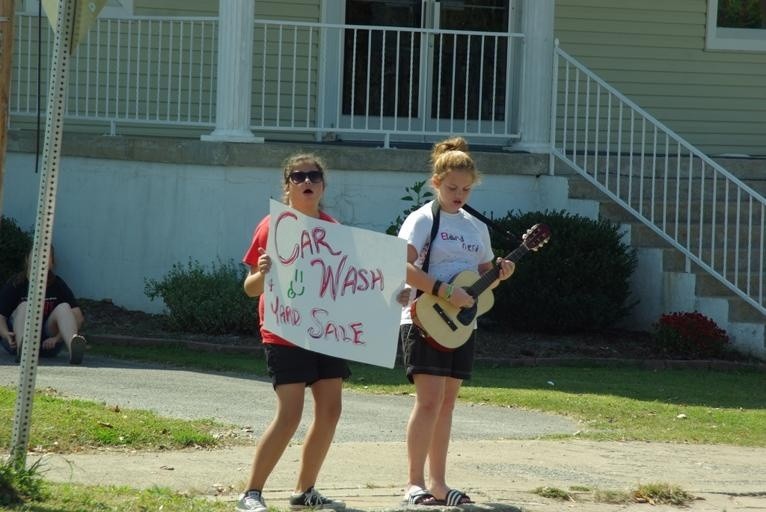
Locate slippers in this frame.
[436,489,475,507]
[402,485,435,507]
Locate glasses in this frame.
[286,171,323,183]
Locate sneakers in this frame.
[289,486,345,510]
[235,489,267,512]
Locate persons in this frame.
[393,136,517,508]
[0,243,88,367]
[232,150,412,512]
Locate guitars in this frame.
[411,223,553,352]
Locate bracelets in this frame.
[430,279,445,298]
[443,284,455,301]
